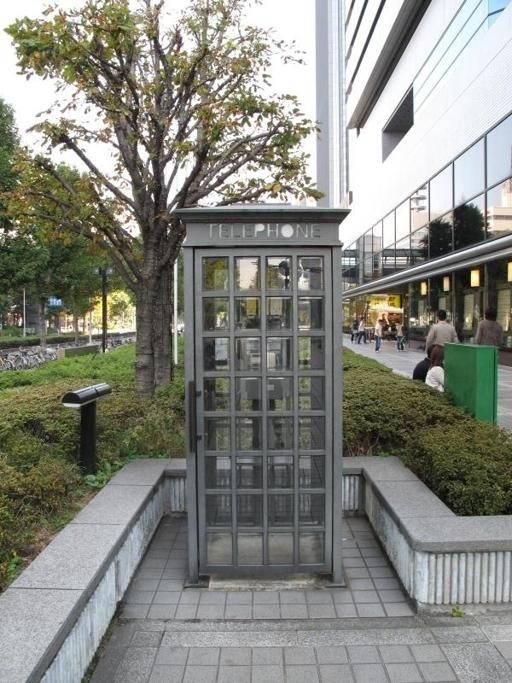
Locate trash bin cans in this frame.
[444,342,499,426]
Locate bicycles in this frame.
[0,332,137,372]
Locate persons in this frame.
[474,308,503,350]
[413,344,434,381]
[425,310,460,352]
[425,345,444,393]
[349,313,406,353]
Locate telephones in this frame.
[237,338,291,399]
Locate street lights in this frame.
[90,265,114,356]
[277,258,293,370]
